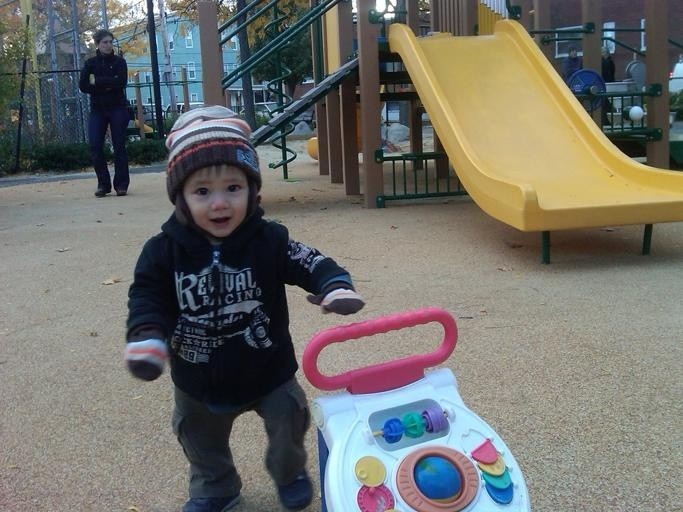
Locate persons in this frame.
[562,43,583,84]
[77,30,130,197]
[600,47,616,85]
[124,104,368,511]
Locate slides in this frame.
[390,18,683,231]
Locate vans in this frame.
[127,102,205,131]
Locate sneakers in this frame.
[277,469,313,512]
[181,483,240,512]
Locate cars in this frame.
[238,101,286,120]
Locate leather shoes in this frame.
[115,189,127,197]
[94,185,111,196]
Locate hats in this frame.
[165,103,262,205]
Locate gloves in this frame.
[124,328,177,382]
[306,280,366,315]
